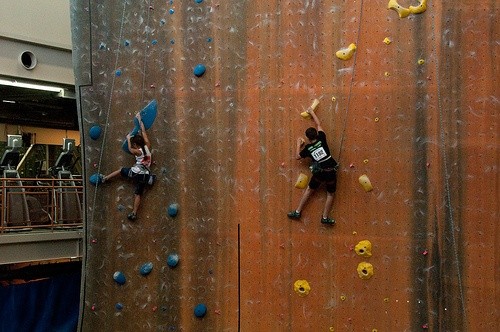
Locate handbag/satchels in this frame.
[148,175,153,185]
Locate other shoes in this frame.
[287,209,301,218]
[127,212,137,221]
[99,173,107,183]
[321,216,335,224]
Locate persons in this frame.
[100,111,151,221]
[287,107,337,223]
